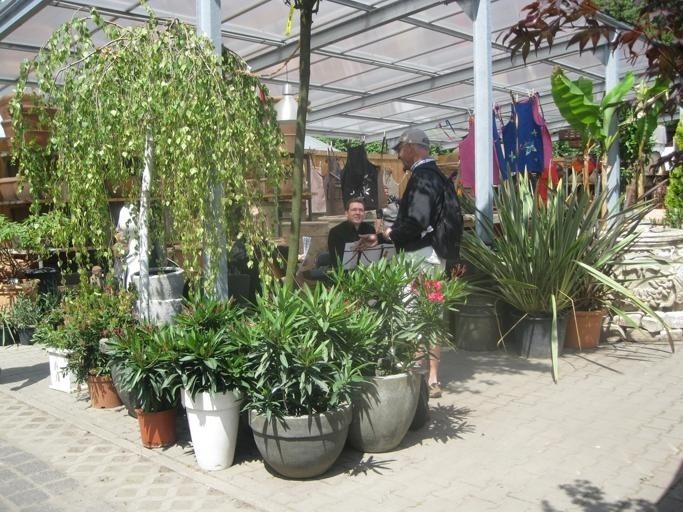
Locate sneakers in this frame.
[426,383,442,397]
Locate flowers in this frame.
[406,258,470,345]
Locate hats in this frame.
[391,129,429,150]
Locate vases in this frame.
[2,93,309,207]
[409,339,441,390]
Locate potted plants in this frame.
[454,170,674,383]
[0,251,422,481]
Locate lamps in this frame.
[273,61,299,126]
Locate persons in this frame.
[228,206,305,313]
[384,189,401,207]
[354,128,464,398]
[328,198,377,273]
